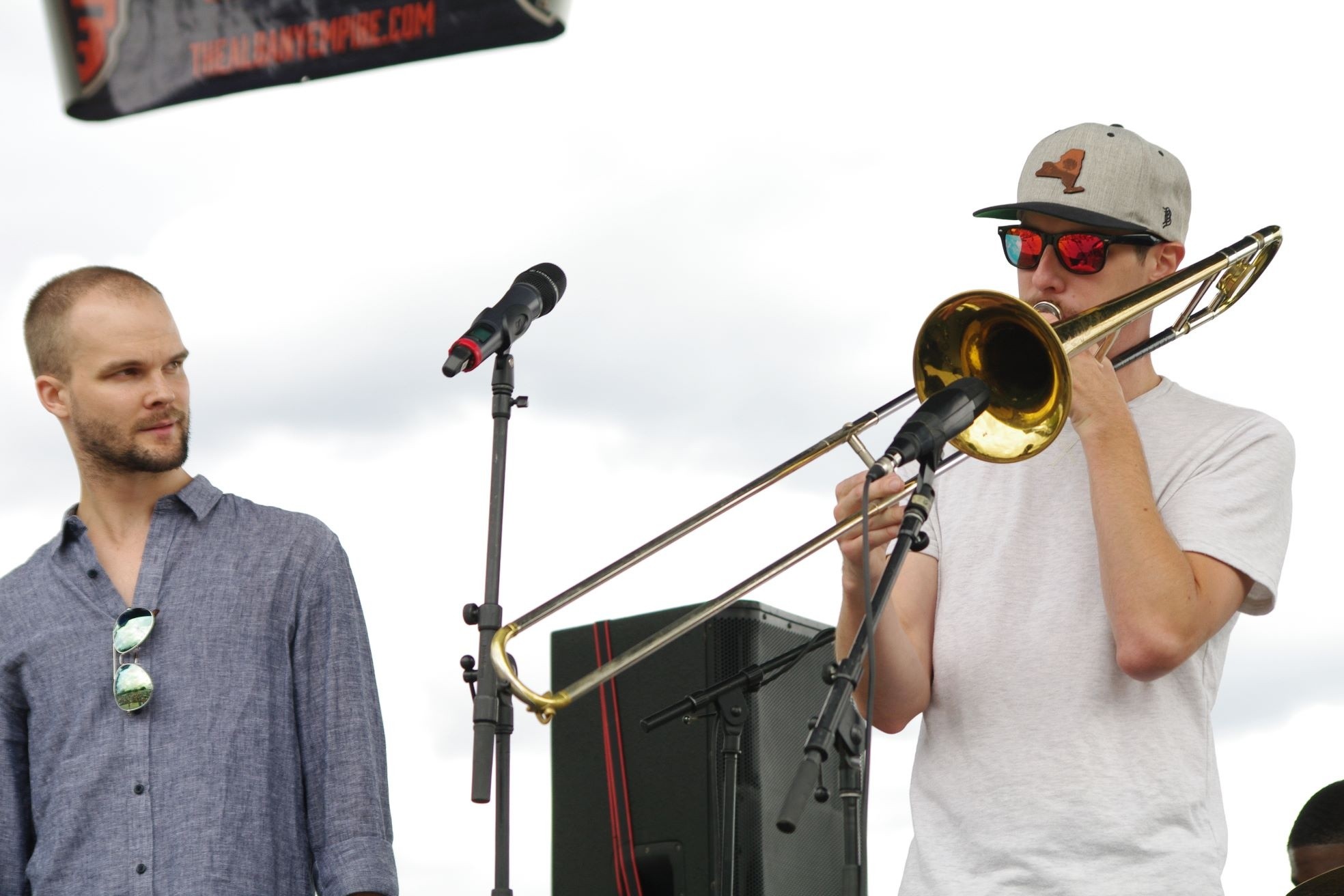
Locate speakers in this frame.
[550,599,868,896]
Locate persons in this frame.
[834,122,1293,896]
[0,266,398,896]
[1286,780,1344,887]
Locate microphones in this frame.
[867,376,993,482]
[441,263,567,378]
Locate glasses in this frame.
[111,606,160,712]
[998,226,1159,275]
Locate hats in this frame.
[973,123,1190,244]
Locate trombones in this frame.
[490,225,1283,722]
